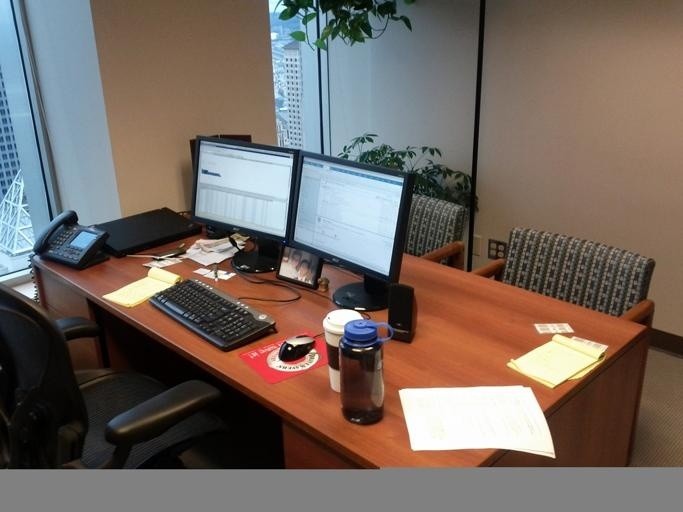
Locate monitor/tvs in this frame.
[288,151,414,312]
[191,135,300,274]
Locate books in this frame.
[102,267,183,307]
[505,333,605,389]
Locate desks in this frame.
[29,251,650,468]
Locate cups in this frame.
[323,308,364,394]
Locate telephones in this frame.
[34,210,109,268]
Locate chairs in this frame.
[0,281,245,468]
[401,192,655,335]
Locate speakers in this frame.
[388,284,417,342]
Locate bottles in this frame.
[338,319,394,426]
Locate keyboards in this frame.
[149,277,277,352]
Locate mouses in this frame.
[279,335,316,361]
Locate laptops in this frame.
[88,207,202,258]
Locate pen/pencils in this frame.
[214,263,218,282]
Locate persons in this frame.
[279,250,312,286]
